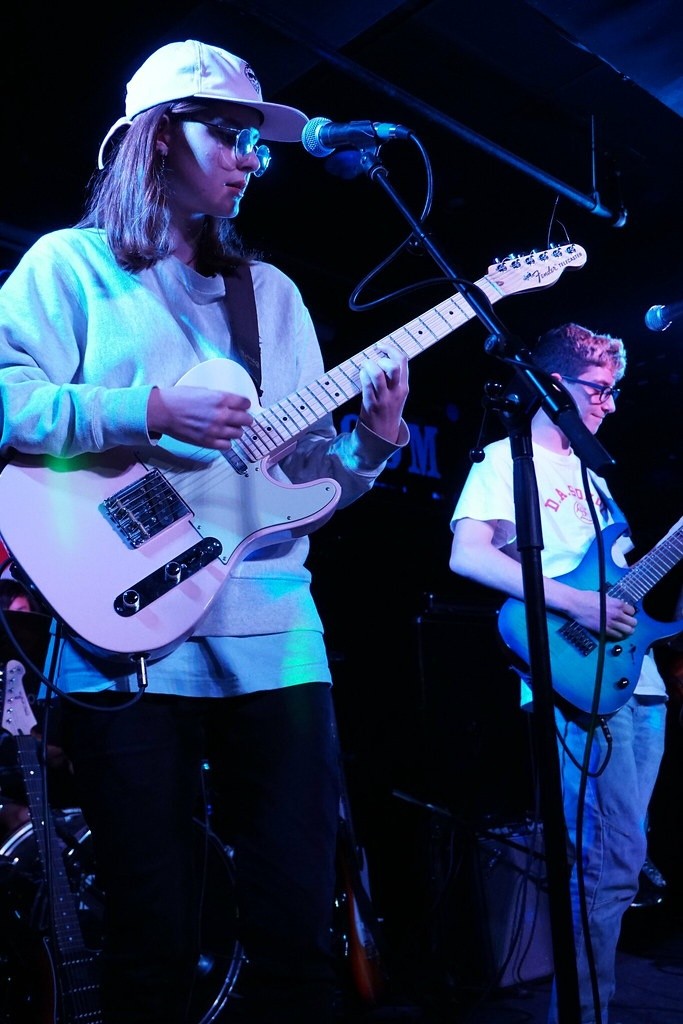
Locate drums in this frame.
[0,803,246,1024]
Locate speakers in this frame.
[438,817,555,992]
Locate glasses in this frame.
[561,374,622,403]
[179,115,272,176]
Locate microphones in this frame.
[645,301,683,331]
[301,116,416,158]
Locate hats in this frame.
[124,38,310,142]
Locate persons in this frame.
[0,39,410,1024]
[448,323,669,1024]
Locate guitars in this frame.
[0,243,588,663]
[498,521,683,717]
[0,659,108,1024]
